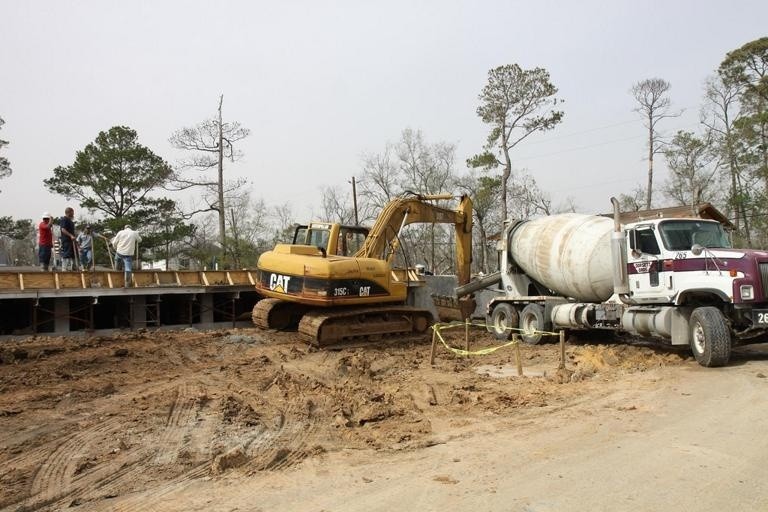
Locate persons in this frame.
[60,207,76,271]
[38,212,53,273]
[76,225,108,272]
[112,224,143,289]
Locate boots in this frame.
[125,271,133,288]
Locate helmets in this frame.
[42,213,51,218]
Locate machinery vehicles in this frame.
[252,190,476,349]
[455,196,768,367]
